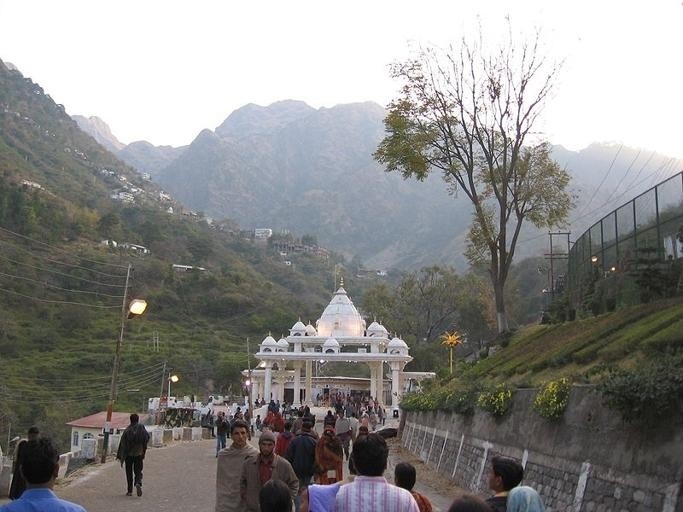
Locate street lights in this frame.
[5,422,19,457]
[154,375,179,424]
[99,262,148,463]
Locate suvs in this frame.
[257,409,315,433]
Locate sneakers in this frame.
[126,492,133,496]
[135,484,142,497]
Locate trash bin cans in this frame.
[393,409,399,418]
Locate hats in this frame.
[259,430,277,443]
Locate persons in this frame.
[8,427,39,501]
[116,415,150,496]
[200,394,545,512]
[0,438,88,512]
